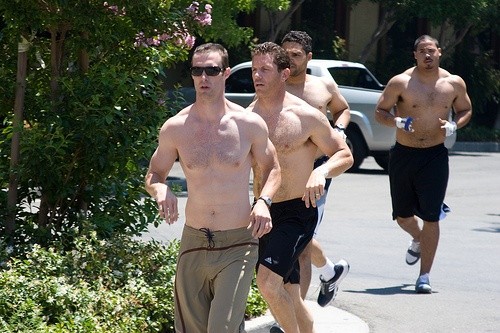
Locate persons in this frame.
[144,44,281,333]
[375,35,472,294]
[274,30,351,333]
[242,43,353,333]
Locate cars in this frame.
[225,58,458,174]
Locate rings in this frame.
[315,193,320,196]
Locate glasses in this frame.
[189,66,226,76]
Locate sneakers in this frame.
[415,282,431,292]
[317,260,349,307]
[405,239,421,265]
[270,322,284,333]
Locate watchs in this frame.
[333,123,346,131]
[259,196,272,206]
